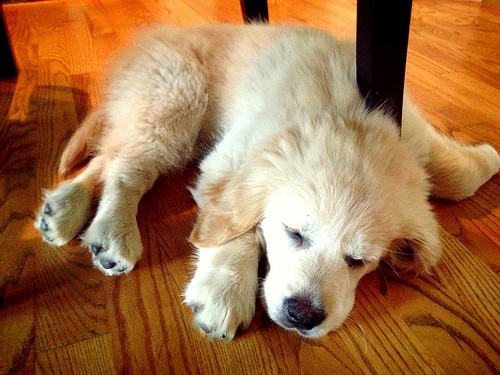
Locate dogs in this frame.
[36,22,500,340]
